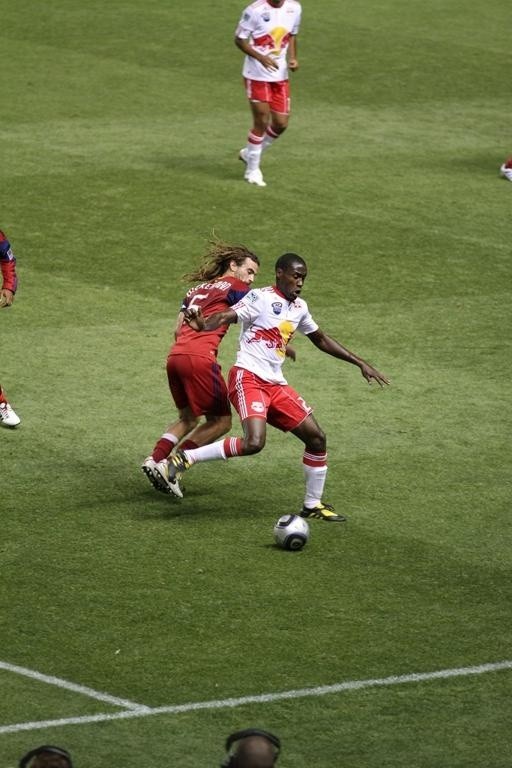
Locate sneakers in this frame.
[298,501,348,523]
[498,162,512,184]
[238,147,251,165]
[0,399,22,430]
[242,167,268,188]
[140,448,192,500]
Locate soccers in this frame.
[274,515,309,551]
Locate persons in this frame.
[500,157,512,181]
[221,726,281,768]
[18,746,73,768]
[0,228,21,427]
[166,252,391,522]
[234,0,302,187]
[142,227,296,501]
